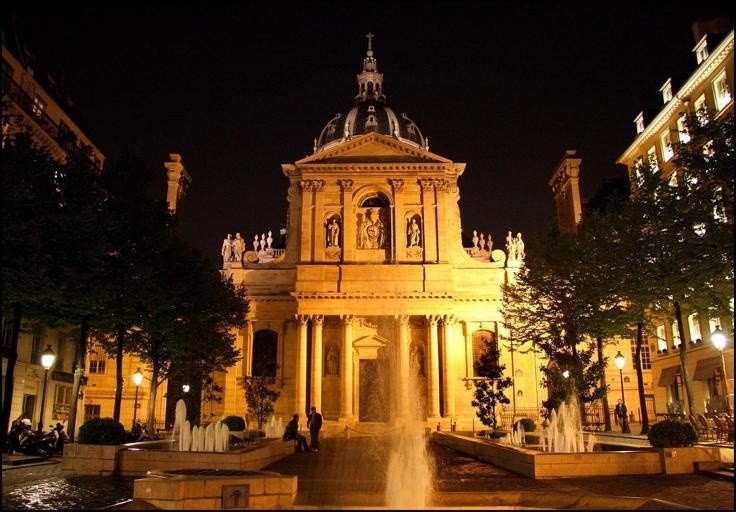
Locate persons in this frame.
[221,233,234,262]
[614,398,627,433]
[227,232,246,261]
[327,218,340,247]
[512,231,526,261]
[306,406,323,453]
[407,218,421,247]
[504,230,518,262]
[283,414,310,452]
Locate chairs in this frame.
[655,410,734,448]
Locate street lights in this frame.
[129,367,142,429]
[562,366,570,386]
[35,342,56,432]
[614,350,631,435]
[181,380,191,411]
[711,325,733,416]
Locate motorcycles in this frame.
[4,412,70,459]
[126,421,152,443]
